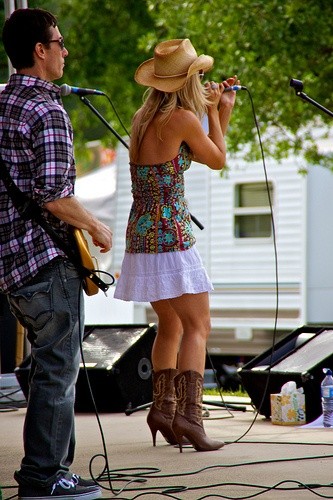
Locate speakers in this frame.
[14,321,158,413]
[238,324,333,424]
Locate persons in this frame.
[0,8,113,500]
[114,38,240,453]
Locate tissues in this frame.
[270,381,306,425]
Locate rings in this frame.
[211,83,218,89]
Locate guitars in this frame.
[75,227,99,296]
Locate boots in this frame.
[171,370,225,453]
[146,368,190,447]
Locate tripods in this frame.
[125,400,246,416]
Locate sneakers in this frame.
[17,474,102,500]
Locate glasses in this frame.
[199,70,204,79]
[50,38,65,49]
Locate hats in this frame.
[135,38,214,92]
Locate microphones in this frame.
[224,85,247,92]
[59,84,105,96]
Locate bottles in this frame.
[320,368,333,428]
